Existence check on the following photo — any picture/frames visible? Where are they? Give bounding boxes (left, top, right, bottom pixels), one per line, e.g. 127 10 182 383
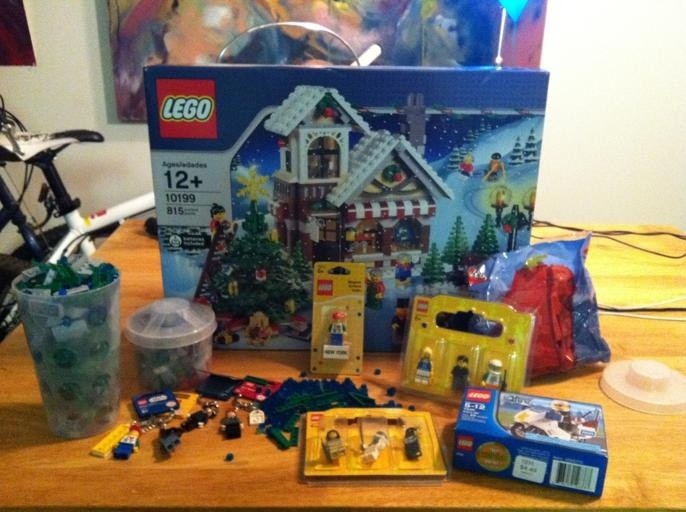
85 0 558 143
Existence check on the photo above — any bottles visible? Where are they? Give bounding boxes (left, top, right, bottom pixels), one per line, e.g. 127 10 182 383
127 295 217 392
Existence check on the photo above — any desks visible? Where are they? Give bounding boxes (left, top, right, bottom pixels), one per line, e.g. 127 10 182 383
0 215 685 511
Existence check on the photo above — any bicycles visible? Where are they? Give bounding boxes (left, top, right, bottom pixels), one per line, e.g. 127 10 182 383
0 21 383 343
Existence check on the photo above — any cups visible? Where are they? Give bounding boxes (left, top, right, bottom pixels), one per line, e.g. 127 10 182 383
8 261 122 438
